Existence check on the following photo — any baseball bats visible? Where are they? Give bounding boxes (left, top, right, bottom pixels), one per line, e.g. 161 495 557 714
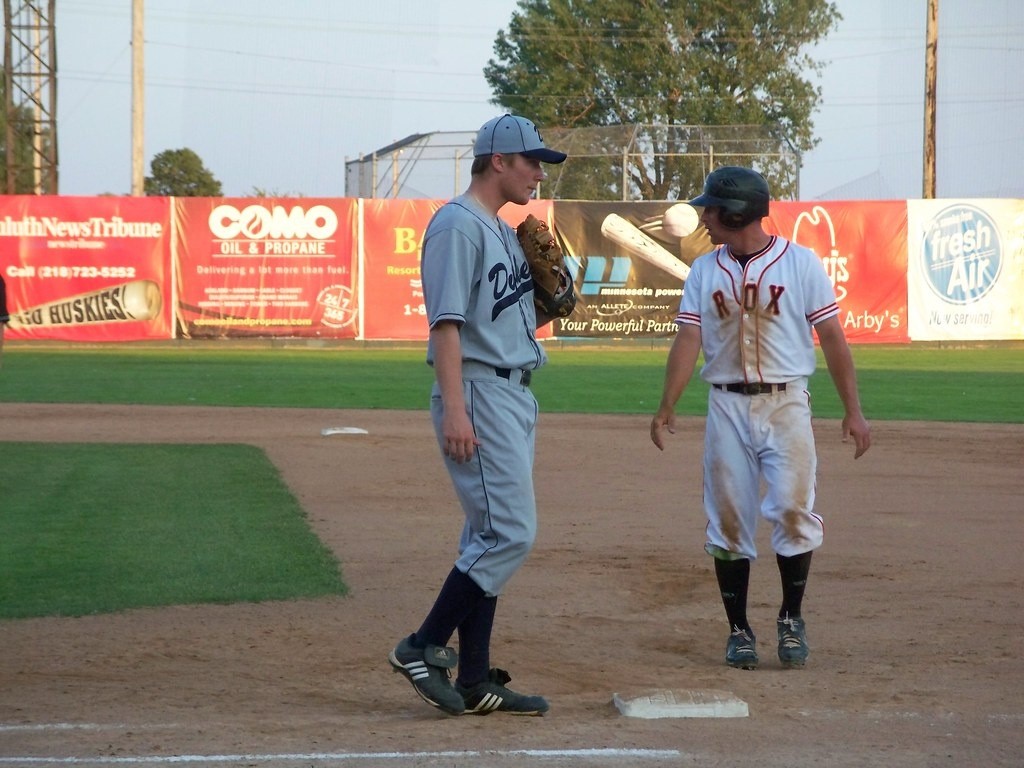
600 213 691 281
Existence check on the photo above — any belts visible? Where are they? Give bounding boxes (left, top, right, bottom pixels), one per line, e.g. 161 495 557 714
491 365 535 389
710 382 788 397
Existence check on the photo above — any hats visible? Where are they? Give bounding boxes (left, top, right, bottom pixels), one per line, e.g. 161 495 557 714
471 114 570 165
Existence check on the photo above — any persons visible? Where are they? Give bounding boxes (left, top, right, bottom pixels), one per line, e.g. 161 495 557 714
648 167 873 668
388 116 577 714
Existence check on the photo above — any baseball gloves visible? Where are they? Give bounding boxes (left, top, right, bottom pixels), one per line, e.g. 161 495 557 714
516 212 577 317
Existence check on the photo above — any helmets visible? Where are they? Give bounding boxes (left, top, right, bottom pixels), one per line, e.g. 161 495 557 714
686 166 774 228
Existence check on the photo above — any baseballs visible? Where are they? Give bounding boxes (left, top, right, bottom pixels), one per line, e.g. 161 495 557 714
662 202 699 237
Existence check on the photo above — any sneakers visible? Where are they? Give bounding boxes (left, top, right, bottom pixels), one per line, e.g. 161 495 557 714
449 666 552 715
724 623 769 671
387 633 488 713
774 611 810 663
